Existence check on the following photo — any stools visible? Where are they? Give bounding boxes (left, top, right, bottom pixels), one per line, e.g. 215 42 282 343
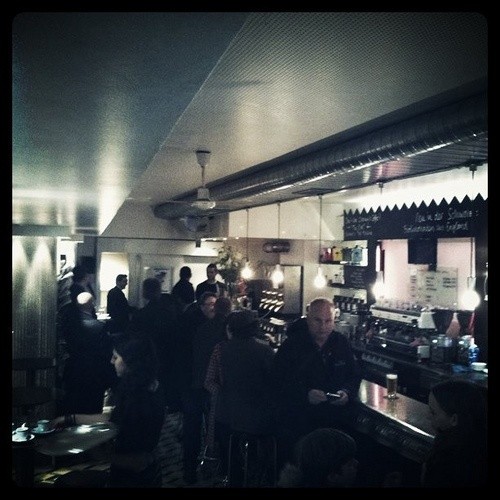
227 432 278 489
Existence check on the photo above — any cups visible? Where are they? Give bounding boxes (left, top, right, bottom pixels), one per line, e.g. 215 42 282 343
386 374 398 399
436 337 449 362
37 420 50 431
12 427 29 440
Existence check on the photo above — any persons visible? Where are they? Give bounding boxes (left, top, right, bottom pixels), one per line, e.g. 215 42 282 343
47 333 164 486
65 265 105 334
61 291 112 414
107 261 487 489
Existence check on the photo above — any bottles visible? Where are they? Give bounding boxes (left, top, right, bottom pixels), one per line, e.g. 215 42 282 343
357 300 364 315
380 319 394 340
333 295 342 309
361 318 380 336
346 297 353 312
394 321 417 344
351 298 359 315
341 297 348 312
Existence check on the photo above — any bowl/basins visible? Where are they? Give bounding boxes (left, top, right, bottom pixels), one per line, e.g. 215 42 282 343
472 362 487 371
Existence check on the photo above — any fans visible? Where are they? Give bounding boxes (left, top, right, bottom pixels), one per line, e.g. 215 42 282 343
123 150 257 212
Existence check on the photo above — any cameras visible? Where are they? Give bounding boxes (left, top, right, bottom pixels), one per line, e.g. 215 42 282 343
325 393 341 400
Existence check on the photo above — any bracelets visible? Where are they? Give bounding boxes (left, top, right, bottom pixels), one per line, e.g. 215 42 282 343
65 413 78 426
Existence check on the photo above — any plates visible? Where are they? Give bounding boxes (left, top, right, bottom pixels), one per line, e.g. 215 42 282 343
12 434 35 442
31 427 56 434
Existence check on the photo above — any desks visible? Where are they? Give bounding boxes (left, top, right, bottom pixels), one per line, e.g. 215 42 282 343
13 357 61 407
11 423 119 487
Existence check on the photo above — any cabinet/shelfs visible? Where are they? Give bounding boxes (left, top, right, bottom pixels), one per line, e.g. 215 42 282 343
317 259 361 288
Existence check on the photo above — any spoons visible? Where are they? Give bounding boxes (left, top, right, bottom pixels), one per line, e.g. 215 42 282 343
26 434 31 442
